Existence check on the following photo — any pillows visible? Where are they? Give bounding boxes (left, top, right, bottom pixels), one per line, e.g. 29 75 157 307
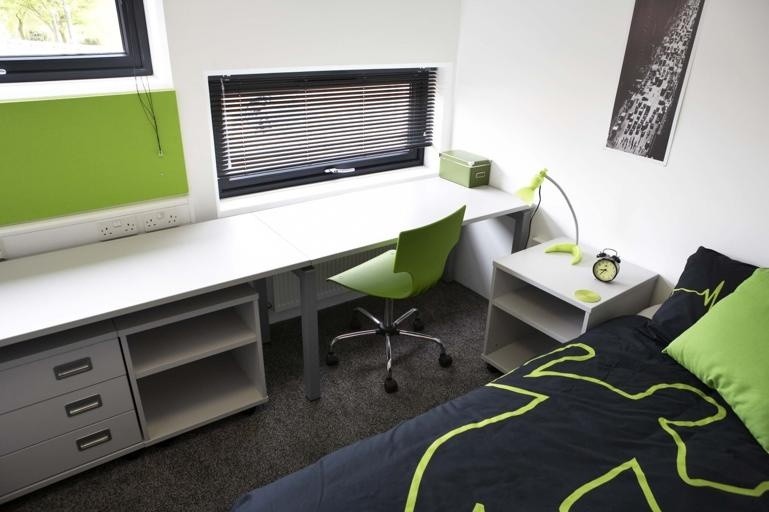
662 267 768 456
645 249 755 344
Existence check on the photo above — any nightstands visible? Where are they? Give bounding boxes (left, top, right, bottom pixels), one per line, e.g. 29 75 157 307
481 237 659 374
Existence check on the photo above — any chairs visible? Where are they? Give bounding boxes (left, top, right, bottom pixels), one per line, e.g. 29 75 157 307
327 204 466 394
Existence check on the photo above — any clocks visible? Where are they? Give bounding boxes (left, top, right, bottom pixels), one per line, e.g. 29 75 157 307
593 248 620 283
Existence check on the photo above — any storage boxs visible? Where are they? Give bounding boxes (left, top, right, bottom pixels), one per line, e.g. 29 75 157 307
439 150 490 188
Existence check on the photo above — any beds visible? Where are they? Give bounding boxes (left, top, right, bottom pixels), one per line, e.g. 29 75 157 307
229 246 769 512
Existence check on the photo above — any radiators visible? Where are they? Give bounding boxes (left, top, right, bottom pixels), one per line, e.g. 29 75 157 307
268 243 397 314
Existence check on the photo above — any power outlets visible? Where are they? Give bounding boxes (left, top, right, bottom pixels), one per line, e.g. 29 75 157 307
98 215 138 242
143 207 181 233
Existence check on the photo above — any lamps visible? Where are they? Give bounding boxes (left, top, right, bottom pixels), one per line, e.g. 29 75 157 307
514 168 582 266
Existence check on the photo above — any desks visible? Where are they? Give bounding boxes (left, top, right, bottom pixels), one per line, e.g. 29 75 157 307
0 176 534 504
